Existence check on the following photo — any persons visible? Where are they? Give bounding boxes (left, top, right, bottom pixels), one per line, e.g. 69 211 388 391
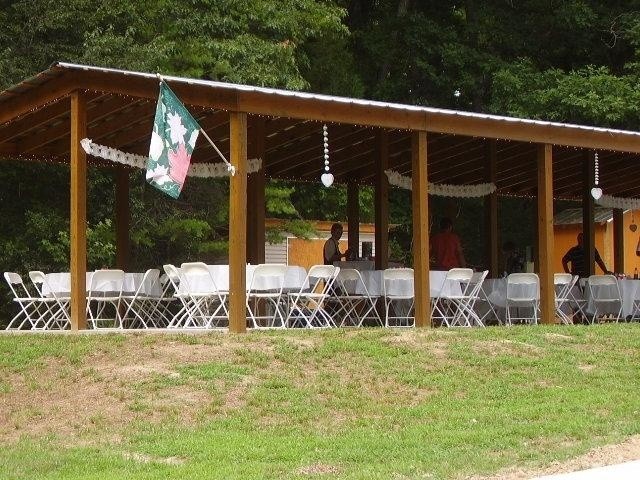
323 223 353 325
503 242 524 276
429 218 468 270
562 233 615 293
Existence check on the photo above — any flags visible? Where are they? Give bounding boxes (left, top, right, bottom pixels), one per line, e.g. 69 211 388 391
146 80 201 200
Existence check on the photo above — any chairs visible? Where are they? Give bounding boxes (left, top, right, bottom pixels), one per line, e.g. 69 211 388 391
246 262 415 328
3 262 73 330
432 269 542 325
85 263 231 329
553 274 638 325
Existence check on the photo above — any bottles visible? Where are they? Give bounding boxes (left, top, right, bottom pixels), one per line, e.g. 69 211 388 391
633 268 638 279
